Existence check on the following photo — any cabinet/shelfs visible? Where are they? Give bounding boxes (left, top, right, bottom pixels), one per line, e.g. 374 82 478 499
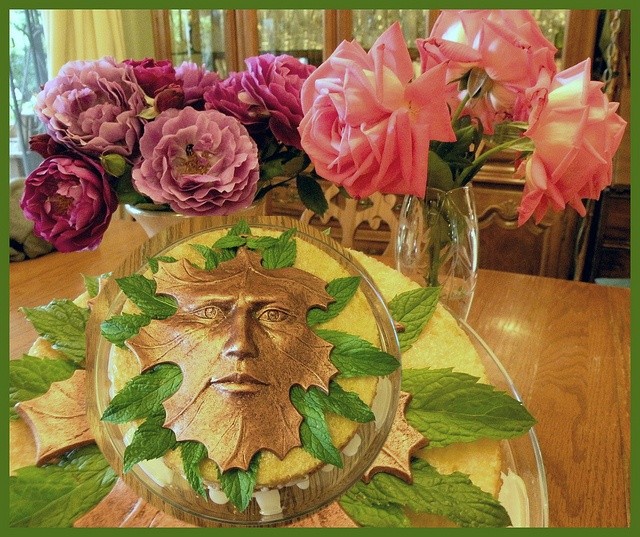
471 183 564 278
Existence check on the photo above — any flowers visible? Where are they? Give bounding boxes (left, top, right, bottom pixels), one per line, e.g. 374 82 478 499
295 10 629 292
18 53 318 254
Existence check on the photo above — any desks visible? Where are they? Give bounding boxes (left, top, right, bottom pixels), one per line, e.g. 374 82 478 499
10 205 629 525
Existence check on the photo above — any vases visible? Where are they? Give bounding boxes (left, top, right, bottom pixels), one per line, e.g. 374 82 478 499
123 202 267 241
395 186 479 328
